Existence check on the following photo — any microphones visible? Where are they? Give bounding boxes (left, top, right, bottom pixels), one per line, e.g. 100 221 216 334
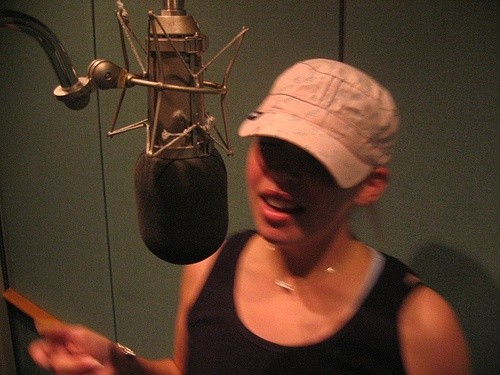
133 0 229 264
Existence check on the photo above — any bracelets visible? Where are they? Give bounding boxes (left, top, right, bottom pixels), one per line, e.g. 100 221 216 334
112 343 138 375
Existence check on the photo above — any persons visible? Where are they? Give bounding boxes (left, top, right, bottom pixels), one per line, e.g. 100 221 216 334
28 59 474 375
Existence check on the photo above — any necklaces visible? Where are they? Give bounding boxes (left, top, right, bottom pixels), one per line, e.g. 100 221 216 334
272 246 359 294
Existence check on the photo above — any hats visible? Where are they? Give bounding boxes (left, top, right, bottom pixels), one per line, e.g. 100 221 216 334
237 58 401 188
